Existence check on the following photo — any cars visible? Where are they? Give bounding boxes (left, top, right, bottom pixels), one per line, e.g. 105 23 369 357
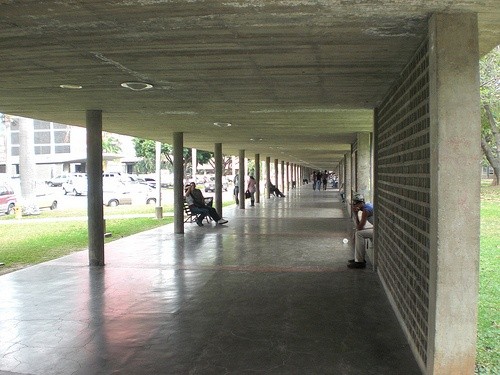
0 181 17 216
204 176 227 192
44 171 157 207
35 183 58 210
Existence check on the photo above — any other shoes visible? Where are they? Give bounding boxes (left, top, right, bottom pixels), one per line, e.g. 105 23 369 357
218 218 229 224
347 258 366 269
194 218 204 226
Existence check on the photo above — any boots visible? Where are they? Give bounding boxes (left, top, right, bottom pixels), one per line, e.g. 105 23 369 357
250 200 254 206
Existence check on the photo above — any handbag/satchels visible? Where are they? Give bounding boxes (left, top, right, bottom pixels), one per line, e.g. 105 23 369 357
245 190 251 199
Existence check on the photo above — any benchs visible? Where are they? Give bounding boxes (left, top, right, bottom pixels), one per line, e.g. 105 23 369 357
351 210 373 265
182 197 213 224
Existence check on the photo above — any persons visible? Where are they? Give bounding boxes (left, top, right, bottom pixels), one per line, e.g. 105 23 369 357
234 174 240 205
329 172 338 187
186 182 228 227
247 176 256 206
347 194 374 268
267 180 285 197
339 186 344 199
313 170 328 190
